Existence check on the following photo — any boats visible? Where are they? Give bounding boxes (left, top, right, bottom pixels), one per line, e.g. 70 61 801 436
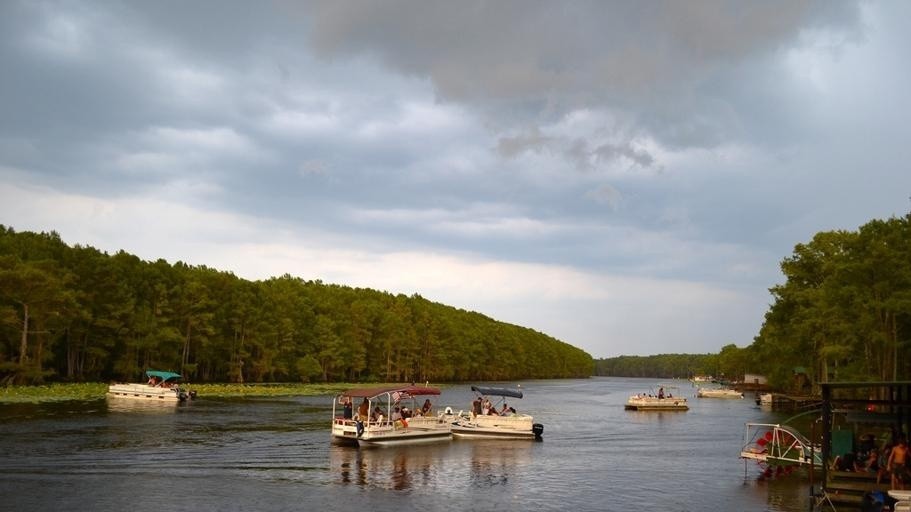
437 385 544 440
625 383 690 412
331 384 454 445
695 387 746 400
107 369 198 401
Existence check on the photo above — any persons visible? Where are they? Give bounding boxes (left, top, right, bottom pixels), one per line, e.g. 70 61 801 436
339 395 519 439
147 375 194 402
659 387 665 399
829 428 911 512
